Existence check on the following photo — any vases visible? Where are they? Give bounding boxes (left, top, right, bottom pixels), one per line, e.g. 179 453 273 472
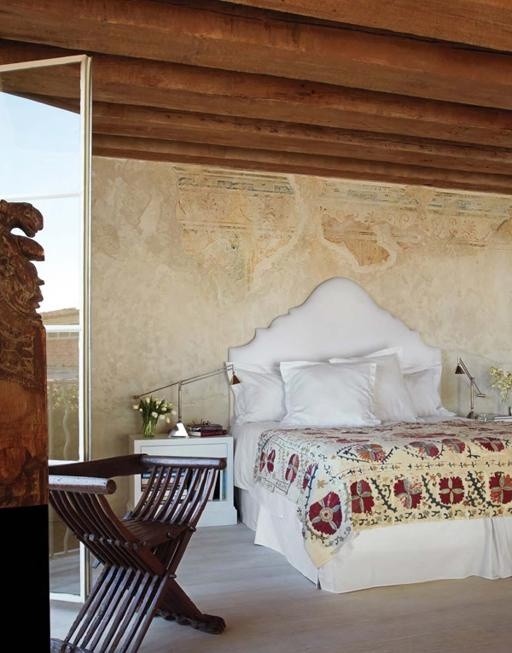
143 417 156 439
497 392 509 416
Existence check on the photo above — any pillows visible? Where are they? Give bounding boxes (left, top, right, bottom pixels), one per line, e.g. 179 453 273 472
277 358 382 429
329 345 418 428
226 362 286 425
402 362 457 424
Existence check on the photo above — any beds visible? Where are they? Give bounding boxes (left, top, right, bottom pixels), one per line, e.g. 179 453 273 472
226 276 512 596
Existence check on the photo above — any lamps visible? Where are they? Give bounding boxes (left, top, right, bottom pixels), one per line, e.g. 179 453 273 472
455 356 486 419
132 364 240 437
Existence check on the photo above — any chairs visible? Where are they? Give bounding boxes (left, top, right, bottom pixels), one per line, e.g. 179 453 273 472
46 452 227 653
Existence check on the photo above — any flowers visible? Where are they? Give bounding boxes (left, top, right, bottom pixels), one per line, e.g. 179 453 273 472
487 365 512 404
131 393 177 439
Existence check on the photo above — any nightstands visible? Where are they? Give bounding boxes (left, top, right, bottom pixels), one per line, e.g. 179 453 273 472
127 433 237 528
479 419 512 423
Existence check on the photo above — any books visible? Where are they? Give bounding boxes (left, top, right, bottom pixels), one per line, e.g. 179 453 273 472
188 425 228 437
142 468 219 505
